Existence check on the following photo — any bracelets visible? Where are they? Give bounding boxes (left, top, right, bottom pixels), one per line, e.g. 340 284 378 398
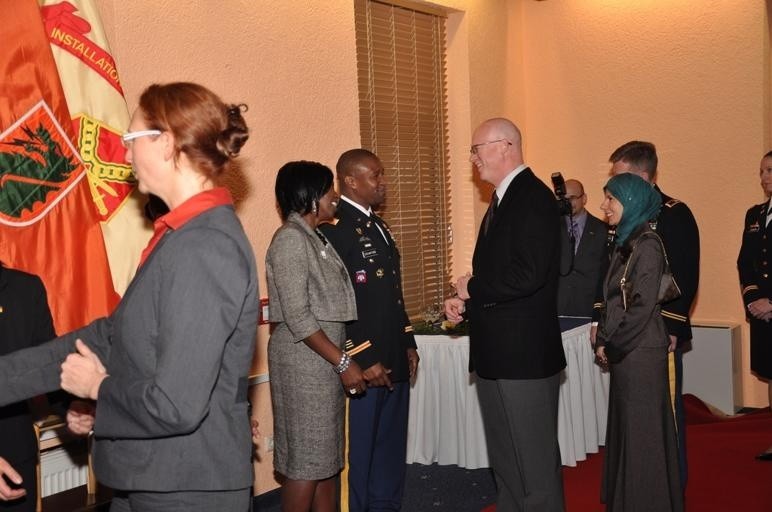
333 352 352 374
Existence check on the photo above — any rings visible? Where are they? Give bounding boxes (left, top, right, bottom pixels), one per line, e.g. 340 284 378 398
349 389 357 395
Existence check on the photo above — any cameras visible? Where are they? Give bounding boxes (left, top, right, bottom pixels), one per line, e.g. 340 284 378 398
551 172 574 215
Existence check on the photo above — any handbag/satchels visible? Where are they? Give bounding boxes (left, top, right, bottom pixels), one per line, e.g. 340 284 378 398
619 231 680 311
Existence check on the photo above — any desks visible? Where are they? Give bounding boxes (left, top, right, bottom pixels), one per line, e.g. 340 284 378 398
406 316 610 470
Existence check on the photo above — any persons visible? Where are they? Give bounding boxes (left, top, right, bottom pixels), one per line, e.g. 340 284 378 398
1 82 260 512
0 262 95 512
556 180 609 317
265 160 367 512
736 150 772 463
319 149 419 512
590 140 700 487
444 118 568 512
594 172 685 511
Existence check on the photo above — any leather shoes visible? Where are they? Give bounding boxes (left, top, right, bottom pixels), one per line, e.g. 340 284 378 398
756 448 772 460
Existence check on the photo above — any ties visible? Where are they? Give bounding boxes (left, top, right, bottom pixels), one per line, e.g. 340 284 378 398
485 191 498 234
369 213 388 246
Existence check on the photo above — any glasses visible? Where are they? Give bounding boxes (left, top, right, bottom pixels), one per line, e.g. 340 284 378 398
470 138 512 155
570 194 583 202
121 130 160 149
